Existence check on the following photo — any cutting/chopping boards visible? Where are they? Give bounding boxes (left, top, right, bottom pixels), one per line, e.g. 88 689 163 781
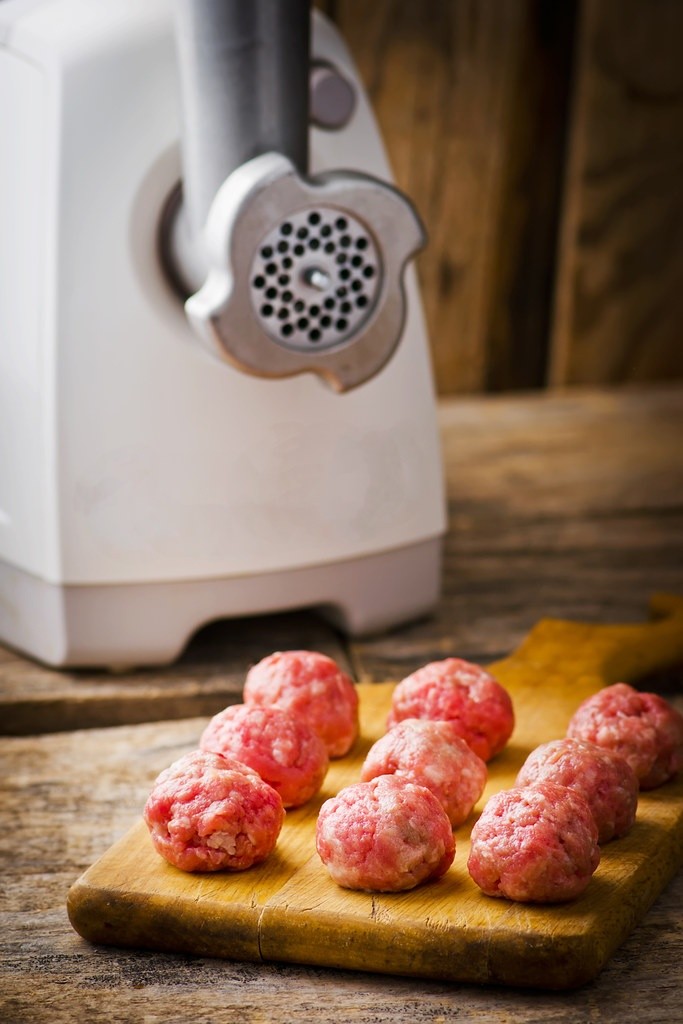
71 591 683 989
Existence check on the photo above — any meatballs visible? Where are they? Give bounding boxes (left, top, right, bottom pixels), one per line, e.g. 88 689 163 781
143 649 683 903
